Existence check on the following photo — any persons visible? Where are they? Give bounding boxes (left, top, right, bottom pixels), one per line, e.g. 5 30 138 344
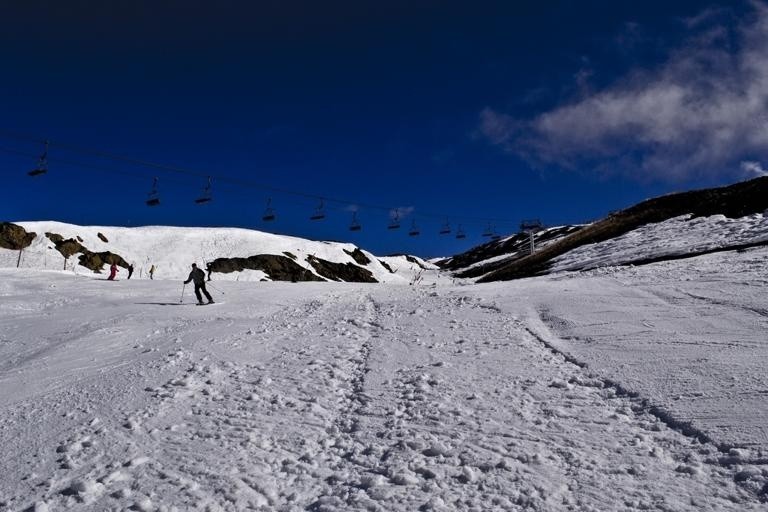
107 261 119 280
127 264 134 279
204 262 212 281
149 264 156 280
183 263 215 305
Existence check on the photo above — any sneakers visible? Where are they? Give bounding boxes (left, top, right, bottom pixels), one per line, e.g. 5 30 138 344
208 299 213 303
199 300 204 304
